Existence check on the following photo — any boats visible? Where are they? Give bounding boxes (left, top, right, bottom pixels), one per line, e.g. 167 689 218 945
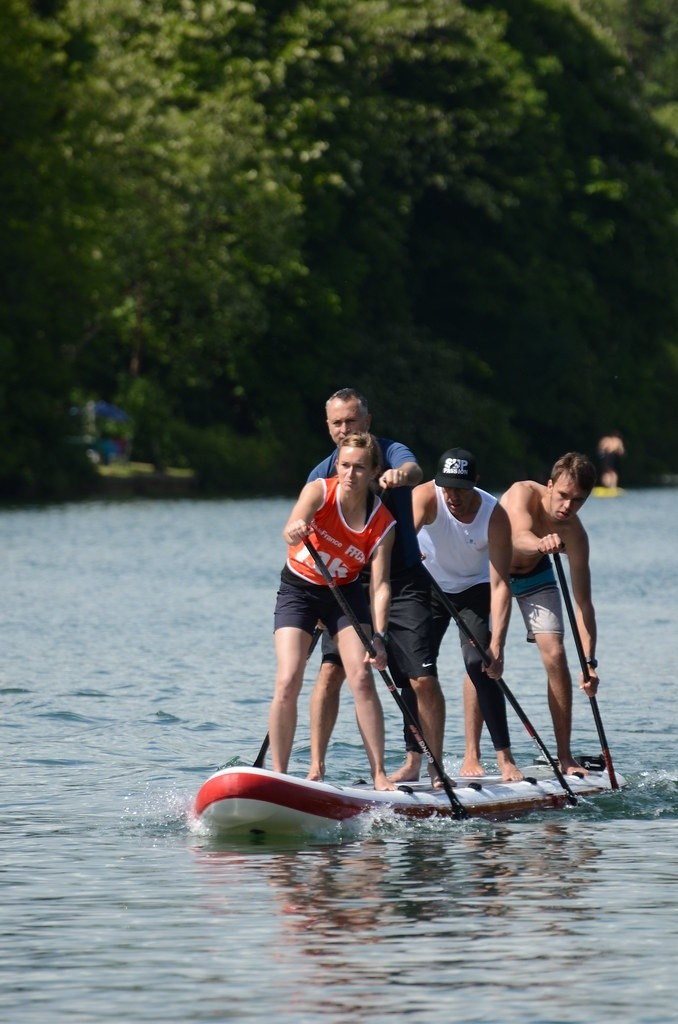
192 755 628 838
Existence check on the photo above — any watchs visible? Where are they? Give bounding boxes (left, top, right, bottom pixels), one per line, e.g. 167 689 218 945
373 633 388 645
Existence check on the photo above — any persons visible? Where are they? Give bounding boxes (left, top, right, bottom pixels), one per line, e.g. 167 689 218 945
388 447 525 782
460 451 599 776
269 432 397 790
600 436 625 488
306 388 457 788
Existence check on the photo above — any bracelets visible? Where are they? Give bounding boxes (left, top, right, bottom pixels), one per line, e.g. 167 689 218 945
585 656 598 669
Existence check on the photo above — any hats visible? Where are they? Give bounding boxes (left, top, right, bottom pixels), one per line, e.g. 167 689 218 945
436 446 477 490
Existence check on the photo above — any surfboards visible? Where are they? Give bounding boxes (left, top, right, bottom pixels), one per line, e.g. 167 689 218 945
195 756 628 827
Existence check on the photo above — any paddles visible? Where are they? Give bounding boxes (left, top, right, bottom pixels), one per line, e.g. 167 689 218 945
419 562 580 807
537 539 618 790
300 533 472 820
253 485 393 768
